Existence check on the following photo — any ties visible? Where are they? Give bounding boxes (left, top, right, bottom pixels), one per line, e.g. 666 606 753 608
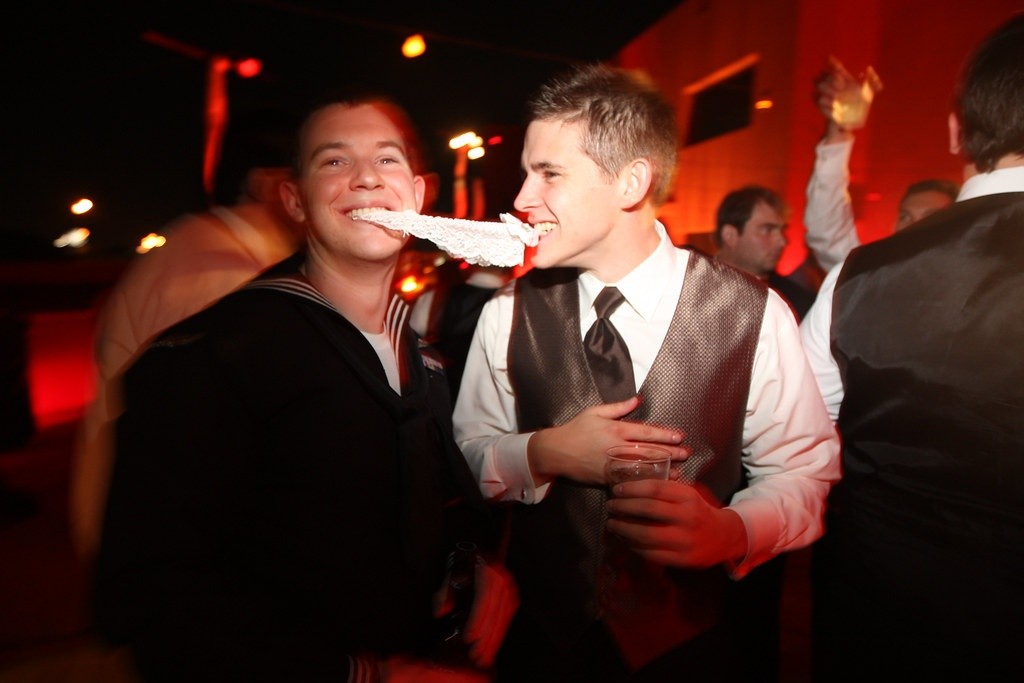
583 287 636 405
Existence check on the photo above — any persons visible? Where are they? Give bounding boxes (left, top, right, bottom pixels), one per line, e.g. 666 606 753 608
809 13 1023 682
62 90 522 683
450 61 844 682
798 63 956 419
712 185 814 323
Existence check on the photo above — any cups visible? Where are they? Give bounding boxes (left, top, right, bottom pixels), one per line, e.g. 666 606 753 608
832 76 875 130
606 445 673 497
